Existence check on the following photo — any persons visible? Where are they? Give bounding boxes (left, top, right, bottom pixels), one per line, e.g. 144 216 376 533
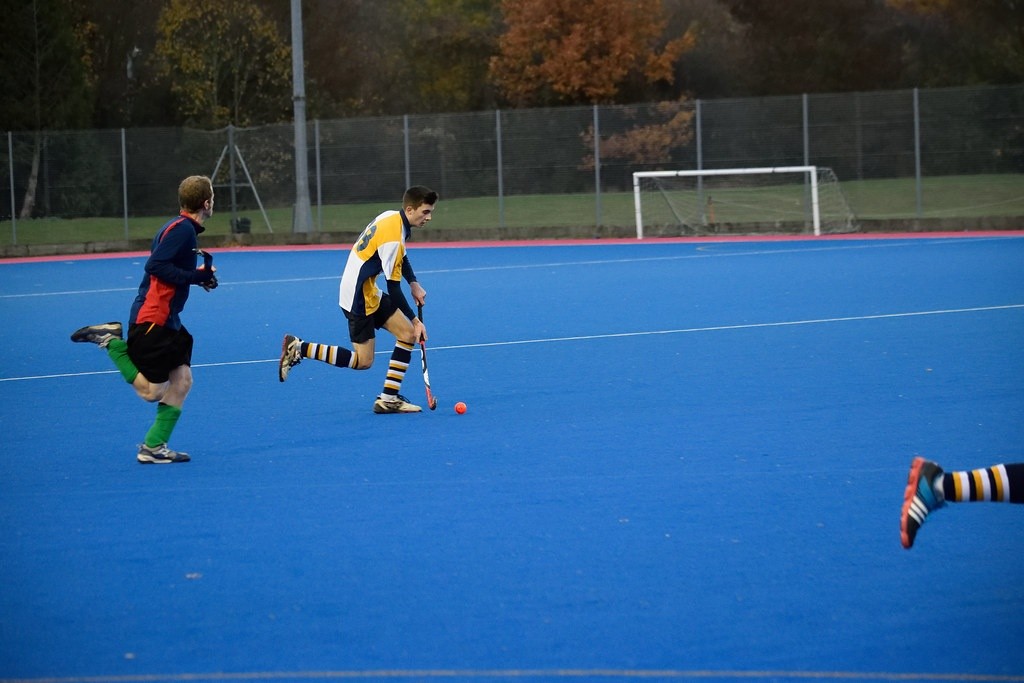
279 185 439 414
899 456 1024 549
70 175 218 463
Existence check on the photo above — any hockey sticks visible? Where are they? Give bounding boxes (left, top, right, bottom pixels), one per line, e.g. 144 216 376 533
416 303 438 410
191 248 214 293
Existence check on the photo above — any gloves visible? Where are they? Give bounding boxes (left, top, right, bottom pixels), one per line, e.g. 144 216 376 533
201 270 219 292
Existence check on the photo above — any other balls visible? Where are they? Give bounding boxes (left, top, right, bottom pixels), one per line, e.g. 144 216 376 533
453 401 468 415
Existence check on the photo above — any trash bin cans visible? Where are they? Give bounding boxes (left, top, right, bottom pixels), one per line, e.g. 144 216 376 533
229 216 251 234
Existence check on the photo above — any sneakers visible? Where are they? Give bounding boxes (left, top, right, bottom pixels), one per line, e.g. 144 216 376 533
71 321 123 349
373 394 422 413
278 334 302 383
900 455 948 550
136 444 190 464
